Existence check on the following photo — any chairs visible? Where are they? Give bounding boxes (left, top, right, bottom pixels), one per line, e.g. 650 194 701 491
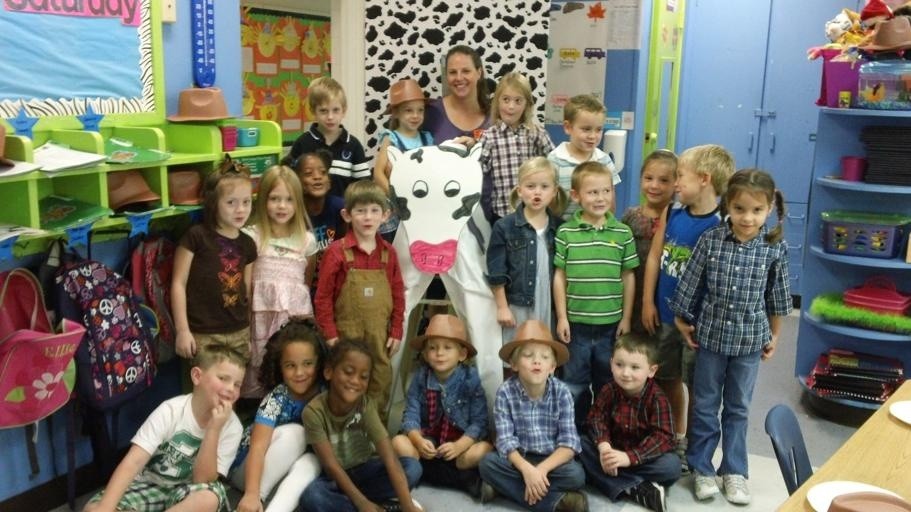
765 404 811 496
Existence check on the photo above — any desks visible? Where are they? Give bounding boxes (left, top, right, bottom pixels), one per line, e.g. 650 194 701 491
774 378 911 512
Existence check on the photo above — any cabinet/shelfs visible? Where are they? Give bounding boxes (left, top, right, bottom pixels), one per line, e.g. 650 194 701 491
794 105 910 410
0 116 284 245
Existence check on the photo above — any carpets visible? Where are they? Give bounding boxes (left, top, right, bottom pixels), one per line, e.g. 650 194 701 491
410 442 817 512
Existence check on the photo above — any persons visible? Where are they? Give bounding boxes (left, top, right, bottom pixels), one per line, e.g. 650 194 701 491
578 330 682 511
480 321 589 511
239 166 319 399
667 168 795 505
287 46 680 425
294 335 424 511
641 144 735 476
84 339 252 511
171 154 257 397
228 314 337 512
392 313 493 497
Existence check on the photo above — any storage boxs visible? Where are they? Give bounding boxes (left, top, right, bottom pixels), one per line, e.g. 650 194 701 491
822 59 868 108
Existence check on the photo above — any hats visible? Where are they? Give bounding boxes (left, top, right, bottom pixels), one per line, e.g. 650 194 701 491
382 80 439 117
105 165 205 218
0 124 15 168
856 17 911 54
164 87 239 122
498 318 571 368
408 312 478 360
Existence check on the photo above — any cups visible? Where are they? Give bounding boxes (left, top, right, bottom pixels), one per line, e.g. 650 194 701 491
843 157 867 181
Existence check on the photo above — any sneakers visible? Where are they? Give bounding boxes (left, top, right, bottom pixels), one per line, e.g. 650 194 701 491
482 484 505 504
724 475 753 506
557 491 591 511
624 477 667 511
693 467 722 502
674 433 689 473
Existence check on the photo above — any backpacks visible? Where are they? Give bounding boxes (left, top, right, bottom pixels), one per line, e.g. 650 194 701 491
1 234 178 430
